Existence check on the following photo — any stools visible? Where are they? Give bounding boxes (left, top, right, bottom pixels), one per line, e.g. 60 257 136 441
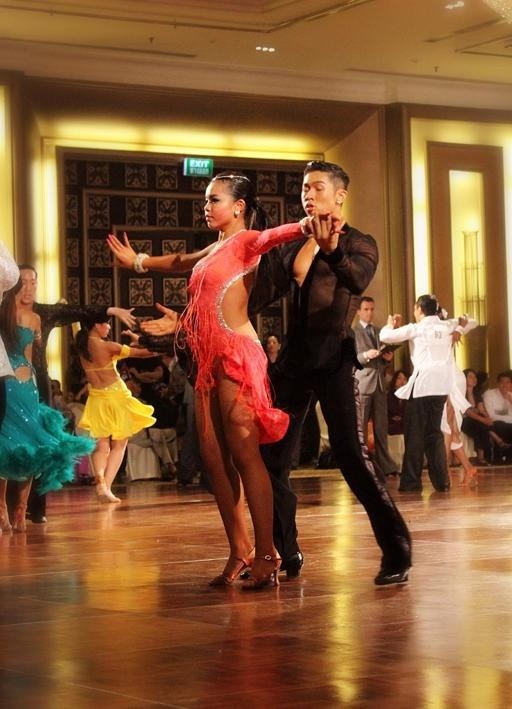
386 432 429 473
458 431 479 460
125 441 164 483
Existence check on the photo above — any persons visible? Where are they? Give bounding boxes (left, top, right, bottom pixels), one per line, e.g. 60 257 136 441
459 369 511 465
261 335 286 392
55 380 90 434
139 161 414 588
378 295 478 493
106 172 345 591
484 372 512 465
1 237 24 437
118 360 138 398
2 265 138 524
2 277 98 533
390 370 410 436
351 296 404 479
397 304 480 488
137 334 169 483
54 298 170 505
169 336 204 484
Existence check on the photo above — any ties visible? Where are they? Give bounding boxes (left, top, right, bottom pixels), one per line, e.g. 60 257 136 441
366 324 376 348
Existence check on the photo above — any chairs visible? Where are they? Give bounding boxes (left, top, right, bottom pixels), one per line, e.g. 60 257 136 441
64 400 113 481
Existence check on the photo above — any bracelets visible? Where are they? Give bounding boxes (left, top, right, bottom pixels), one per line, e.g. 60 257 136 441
134 253 151 275
299 216 317 238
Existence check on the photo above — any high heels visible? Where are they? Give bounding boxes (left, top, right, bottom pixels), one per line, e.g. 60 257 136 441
459 467 479 487
12 504 28 532
450 432 464 451
0 505 13 535
242 552 284 590
210 546 255 586
96 476 122 503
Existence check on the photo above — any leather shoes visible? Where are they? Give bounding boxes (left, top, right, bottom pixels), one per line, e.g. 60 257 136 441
498 441 512 451
31 514 47 523
241 551 304 578
399 487 422 492
375 566 410 585
477 458 491 466
435 481 450 492
